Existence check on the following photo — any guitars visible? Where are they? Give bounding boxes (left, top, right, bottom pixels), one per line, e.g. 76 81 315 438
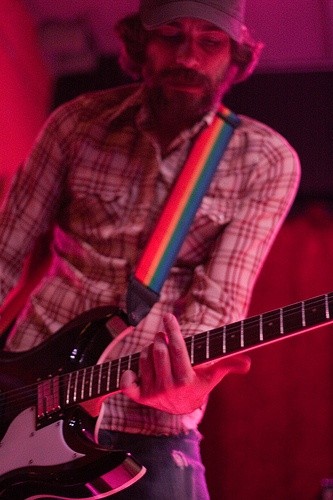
1 292 332 500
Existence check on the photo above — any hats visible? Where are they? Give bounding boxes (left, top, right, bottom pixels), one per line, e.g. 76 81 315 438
137 0 247 42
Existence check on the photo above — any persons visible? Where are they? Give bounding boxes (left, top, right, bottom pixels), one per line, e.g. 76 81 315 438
0 0 305 500
